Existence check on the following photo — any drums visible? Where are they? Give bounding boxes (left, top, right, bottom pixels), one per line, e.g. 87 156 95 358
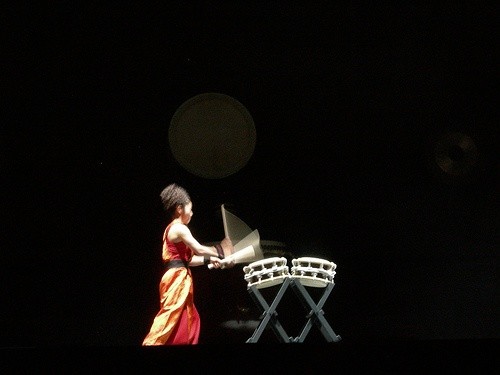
291 256 337 287
244 257 290 288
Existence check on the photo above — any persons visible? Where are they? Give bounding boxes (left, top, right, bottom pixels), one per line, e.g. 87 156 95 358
142 182 233 345
